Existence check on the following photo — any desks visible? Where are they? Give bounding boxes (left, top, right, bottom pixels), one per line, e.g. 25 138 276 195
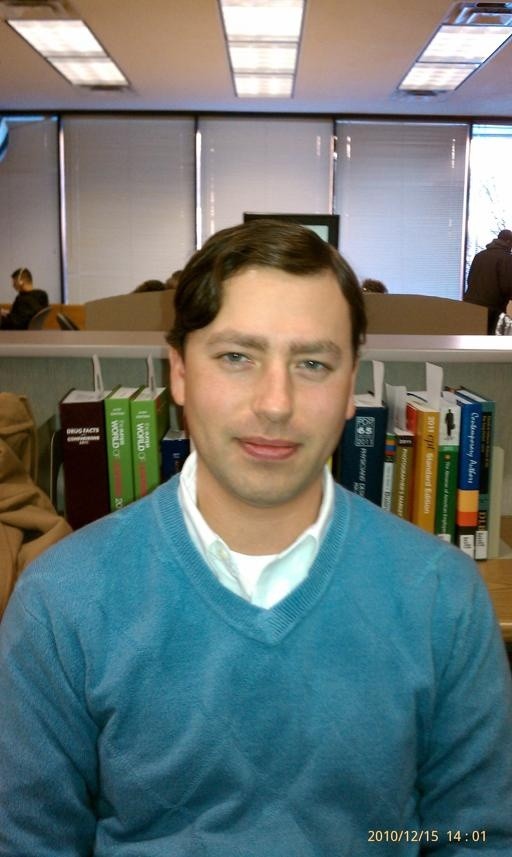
0 331 512 642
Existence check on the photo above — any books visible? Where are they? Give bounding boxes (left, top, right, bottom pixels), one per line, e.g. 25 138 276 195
329 387 494 562
59 384 191 533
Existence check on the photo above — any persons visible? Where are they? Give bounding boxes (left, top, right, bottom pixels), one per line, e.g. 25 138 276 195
1 216 512 856
1 266 52 330
128 269 183 295
363 278 387 294
463 228 512 335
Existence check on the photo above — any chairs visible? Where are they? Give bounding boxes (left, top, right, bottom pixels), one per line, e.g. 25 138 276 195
28 307 53 330
57 312 80 330
